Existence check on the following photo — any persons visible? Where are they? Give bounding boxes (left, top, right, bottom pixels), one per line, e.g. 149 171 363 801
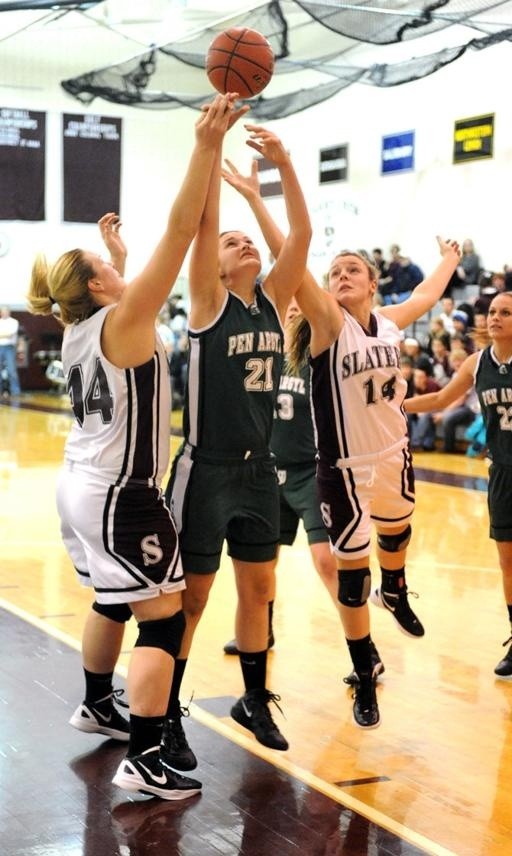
371 234 511 291
160 294 190 407
0 306 26 400
401 292 511 676
403 395 482 453
159 92 317 770
26 90 231 801
222 293 386 685
218 158 463 730
402 292 494 394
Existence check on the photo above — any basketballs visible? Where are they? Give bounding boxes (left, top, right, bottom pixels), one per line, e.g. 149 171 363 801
209 29 276 99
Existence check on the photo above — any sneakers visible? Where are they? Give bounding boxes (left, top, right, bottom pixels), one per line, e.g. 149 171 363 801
156 692 198 771
69 689 131 741
224 630 275 655
343 655 384 686
494 637 511 680
231 689 289 751
112 746 202 801
370 584 424 638
351 673 380 729
